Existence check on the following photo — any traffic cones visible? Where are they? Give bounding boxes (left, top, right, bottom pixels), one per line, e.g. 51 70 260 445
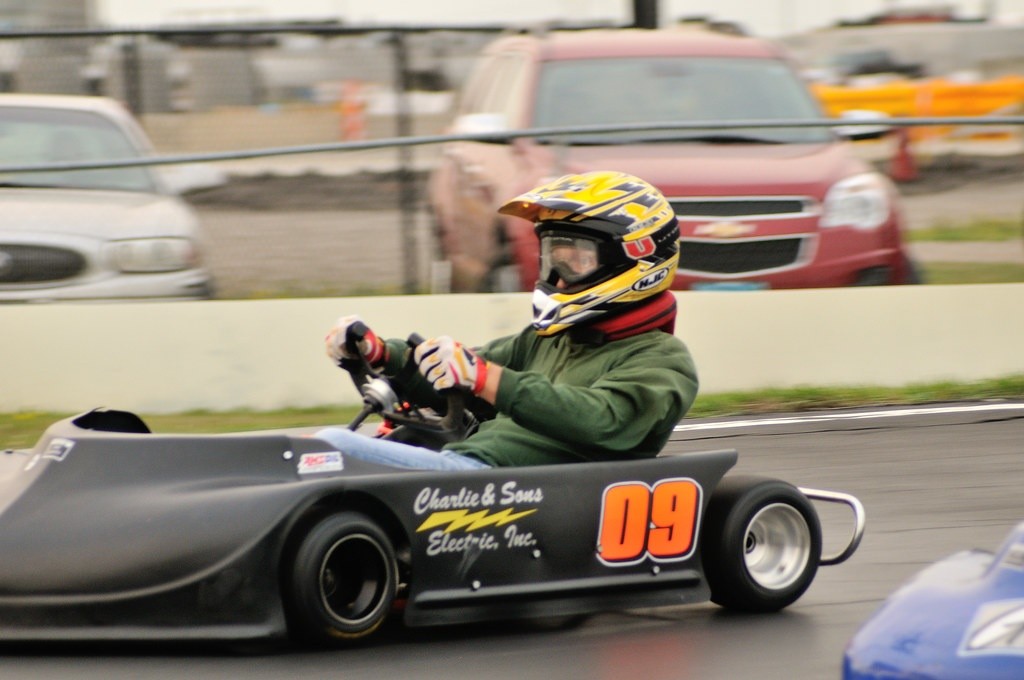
335 75 370 141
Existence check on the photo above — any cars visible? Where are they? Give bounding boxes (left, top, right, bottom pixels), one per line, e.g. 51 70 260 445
1 92 217 304
421 26 920 293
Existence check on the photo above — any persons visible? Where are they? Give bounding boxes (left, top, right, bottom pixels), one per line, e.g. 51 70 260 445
326 172 699 471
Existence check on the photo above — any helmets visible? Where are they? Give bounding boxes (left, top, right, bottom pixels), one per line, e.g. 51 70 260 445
497 170 682 338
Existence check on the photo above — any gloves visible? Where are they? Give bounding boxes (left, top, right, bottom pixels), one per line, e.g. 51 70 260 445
414 335 490 396
324 315 390 372
